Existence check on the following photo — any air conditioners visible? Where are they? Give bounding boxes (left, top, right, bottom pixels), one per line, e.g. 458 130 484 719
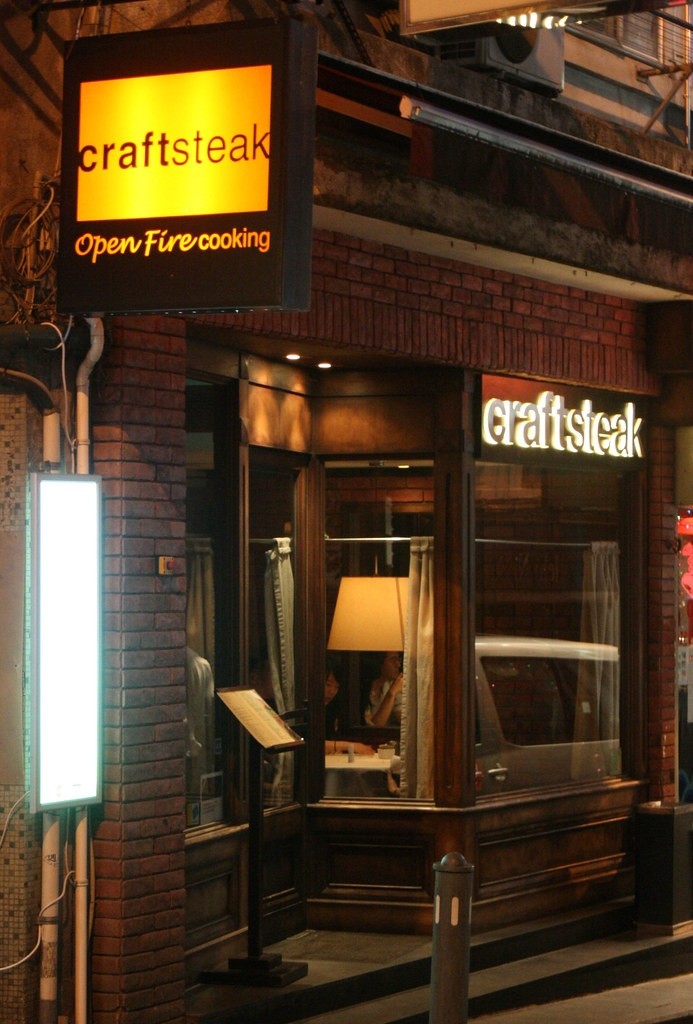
436 24 565 99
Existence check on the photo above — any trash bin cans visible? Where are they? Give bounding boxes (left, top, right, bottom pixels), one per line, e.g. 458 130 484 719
636 799 693 937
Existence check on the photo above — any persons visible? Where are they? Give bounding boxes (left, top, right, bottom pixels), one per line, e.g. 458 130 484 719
324 657 375 756
362 651 403 728
249 657 274 706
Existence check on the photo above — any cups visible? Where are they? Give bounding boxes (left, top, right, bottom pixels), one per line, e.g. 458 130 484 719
348 744 354 754
378 748 395 759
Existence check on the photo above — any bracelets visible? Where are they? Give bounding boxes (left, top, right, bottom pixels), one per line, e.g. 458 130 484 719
334 740 336 752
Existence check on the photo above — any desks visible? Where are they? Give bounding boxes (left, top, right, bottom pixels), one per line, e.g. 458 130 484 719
325 754 400 797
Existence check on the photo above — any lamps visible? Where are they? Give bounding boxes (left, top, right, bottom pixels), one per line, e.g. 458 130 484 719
327 554 409 651
30 471 104 816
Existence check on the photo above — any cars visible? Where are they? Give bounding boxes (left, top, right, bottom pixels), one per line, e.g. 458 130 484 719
473 637 618 796
